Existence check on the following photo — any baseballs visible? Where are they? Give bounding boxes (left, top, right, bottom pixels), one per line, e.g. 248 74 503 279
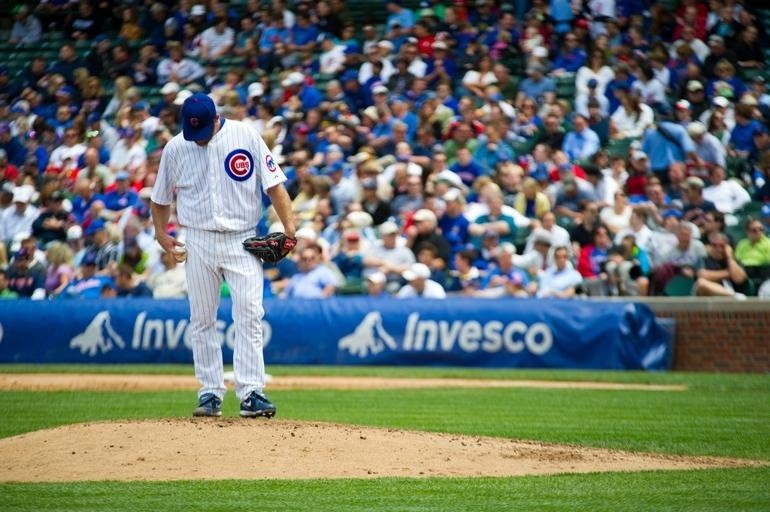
174 248 186 262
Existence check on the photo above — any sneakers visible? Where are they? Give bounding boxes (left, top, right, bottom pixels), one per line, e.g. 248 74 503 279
193 394 223 416
240 391 276 419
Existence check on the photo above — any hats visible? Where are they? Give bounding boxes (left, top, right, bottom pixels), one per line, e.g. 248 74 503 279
0 0 770 301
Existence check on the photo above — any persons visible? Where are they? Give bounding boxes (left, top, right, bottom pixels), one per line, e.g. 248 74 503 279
151 92 297 418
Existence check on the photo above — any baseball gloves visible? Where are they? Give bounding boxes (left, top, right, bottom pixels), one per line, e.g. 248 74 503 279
242 233 297 265
